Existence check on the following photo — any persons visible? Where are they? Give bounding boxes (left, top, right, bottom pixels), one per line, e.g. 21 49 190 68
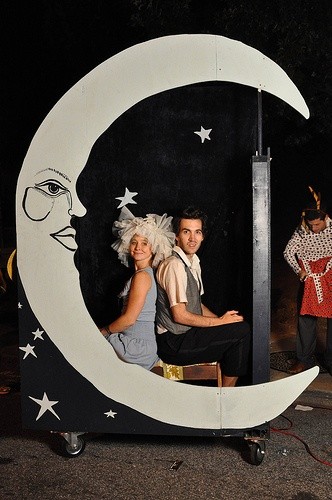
96 205 177 371
283 209 332 374
155 206 253 387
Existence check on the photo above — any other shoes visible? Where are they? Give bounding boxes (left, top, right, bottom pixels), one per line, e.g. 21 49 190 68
289 362 304 374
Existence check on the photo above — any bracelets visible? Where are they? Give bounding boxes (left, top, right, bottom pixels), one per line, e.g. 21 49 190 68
105 327 113 335
